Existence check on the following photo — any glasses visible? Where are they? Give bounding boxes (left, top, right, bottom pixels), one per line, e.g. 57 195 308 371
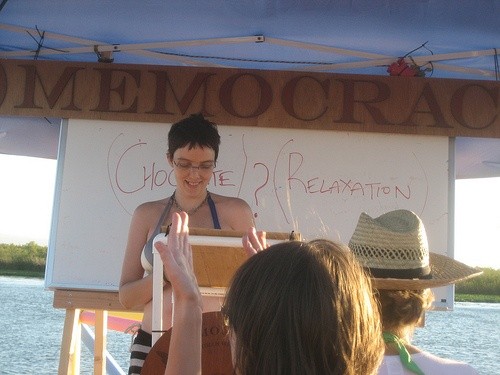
174 161 216 172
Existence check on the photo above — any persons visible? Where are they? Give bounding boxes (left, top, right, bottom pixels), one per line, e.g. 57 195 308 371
154 212 385 374
117 114 260 375
347 209 486 374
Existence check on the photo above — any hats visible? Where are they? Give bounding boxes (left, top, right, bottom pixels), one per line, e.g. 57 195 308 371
348 209 485 290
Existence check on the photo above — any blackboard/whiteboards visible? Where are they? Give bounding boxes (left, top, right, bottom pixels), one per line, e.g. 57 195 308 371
45 115 459 316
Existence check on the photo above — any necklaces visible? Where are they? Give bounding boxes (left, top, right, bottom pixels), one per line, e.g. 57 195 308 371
174 195 208 215
